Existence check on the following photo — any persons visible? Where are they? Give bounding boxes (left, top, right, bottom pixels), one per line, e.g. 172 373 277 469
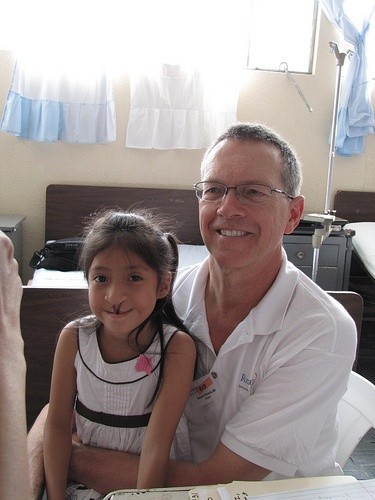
43 212 195 500
26 124 357 500
0 232 31 500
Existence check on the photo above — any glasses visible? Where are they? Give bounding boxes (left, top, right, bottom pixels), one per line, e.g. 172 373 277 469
192 181 295 204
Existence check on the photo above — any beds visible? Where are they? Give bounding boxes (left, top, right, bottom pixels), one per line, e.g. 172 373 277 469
333 191 375 283
20 184 364 435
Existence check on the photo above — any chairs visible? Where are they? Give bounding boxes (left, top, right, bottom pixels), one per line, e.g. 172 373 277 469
261 370 374 480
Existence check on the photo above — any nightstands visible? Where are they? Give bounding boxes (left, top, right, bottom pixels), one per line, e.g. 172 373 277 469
282 227 356 291
0 215 27 279
